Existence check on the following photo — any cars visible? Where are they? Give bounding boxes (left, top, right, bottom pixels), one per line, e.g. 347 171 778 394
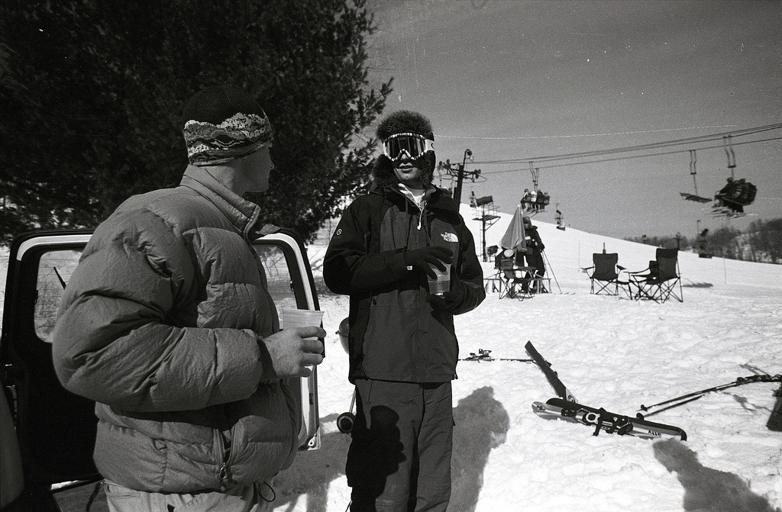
1 226 322 511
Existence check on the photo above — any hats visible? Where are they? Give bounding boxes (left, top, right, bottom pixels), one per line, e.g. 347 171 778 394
182 87 274 166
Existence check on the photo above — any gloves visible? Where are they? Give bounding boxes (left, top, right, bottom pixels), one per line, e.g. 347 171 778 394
429 279 467 312
403 247 453 281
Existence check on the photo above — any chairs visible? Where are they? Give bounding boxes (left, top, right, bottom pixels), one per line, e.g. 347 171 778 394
485 246 683 304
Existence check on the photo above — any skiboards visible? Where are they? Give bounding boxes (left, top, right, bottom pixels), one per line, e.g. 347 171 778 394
518 289 575 296
697 206 758 222
525 209 539 218
525 340 687 443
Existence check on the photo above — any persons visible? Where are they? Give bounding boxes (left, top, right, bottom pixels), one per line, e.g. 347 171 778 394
503 250 532 293
323 111 486 512
711 177 757 217
52 85 326 512
520 188 550 212
521 216 545 293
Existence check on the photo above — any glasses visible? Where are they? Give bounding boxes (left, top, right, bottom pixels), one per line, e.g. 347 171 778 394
382 134 435 161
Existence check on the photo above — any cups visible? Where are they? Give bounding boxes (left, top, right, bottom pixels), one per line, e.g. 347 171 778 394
425 256 452 297
281 308 324 373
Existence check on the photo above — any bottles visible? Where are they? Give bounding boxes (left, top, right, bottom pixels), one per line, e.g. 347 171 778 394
603 242 606 254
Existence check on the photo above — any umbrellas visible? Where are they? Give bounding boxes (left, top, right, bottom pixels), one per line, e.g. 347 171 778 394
500 205 525 252
